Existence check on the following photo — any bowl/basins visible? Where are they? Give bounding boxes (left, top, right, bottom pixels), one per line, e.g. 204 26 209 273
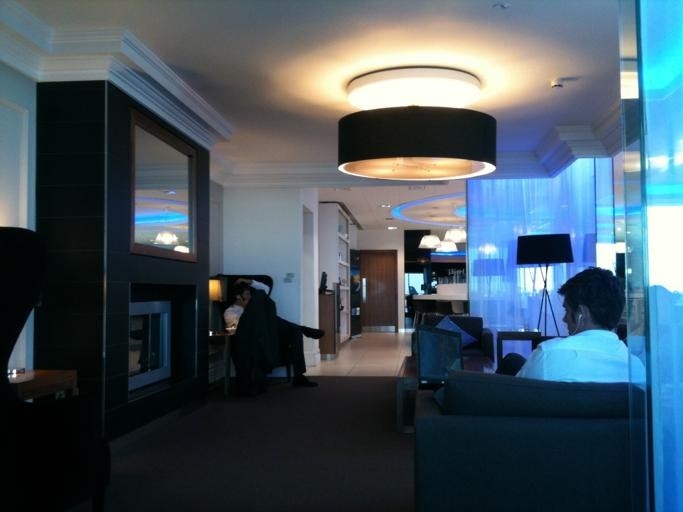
225 327 236 334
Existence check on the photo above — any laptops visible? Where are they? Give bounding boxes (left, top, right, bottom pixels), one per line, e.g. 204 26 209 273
415 323 468 392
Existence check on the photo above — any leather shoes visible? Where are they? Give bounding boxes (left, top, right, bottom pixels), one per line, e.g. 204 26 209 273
292 376 318 387
306 329 324 339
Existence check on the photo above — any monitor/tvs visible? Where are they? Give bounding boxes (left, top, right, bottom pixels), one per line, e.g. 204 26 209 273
319 272 327 292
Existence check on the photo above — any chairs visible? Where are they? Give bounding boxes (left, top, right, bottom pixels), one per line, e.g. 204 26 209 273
209 275 292 397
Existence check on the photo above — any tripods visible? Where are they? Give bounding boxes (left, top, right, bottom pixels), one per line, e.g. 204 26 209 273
533 289 561 340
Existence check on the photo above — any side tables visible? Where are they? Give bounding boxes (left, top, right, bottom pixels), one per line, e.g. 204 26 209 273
1 366 79 405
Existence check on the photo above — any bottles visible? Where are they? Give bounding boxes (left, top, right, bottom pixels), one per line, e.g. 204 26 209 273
431 271 438 294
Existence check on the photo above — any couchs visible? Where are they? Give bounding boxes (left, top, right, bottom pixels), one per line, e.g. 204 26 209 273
413 314 494 367
413 372 652 510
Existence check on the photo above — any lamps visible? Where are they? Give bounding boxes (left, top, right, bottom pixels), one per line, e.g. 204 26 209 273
515 234 574 336
417 228 467 253
208 279 223 303
336 66 496 182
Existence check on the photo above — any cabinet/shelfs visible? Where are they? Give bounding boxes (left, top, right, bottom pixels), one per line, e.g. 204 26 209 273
319 202 351 346
319 283 341 359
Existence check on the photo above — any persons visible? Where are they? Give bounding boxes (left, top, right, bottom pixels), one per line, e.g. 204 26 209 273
501 267 644 382
222 281 324 388
